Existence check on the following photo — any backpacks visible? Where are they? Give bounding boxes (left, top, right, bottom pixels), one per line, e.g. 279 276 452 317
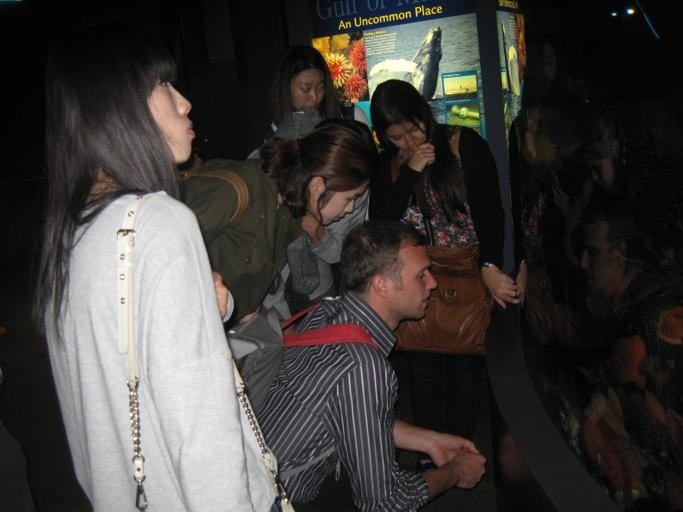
226 298 382 413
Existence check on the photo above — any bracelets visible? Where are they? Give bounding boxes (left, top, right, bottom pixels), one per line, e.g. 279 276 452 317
482 260 495 267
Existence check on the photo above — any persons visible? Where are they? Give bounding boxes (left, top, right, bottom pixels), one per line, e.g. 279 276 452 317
372 79 523 474
30 23 296 512
248 107 371 314
259 43 369 148
179 116 381 331
255 215 486 512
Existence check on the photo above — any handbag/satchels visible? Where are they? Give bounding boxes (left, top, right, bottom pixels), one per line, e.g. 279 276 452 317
393 243 498 357
